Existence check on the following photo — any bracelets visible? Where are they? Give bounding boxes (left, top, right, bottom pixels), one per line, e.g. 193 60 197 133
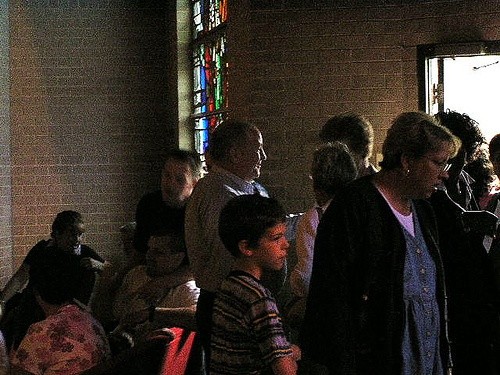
148 305 156 322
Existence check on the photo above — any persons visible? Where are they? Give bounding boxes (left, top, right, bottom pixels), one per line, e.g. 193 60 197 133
185 118 267 375
319 112 378 177
0 211 111 353
102 228 201 355
301 111 461 375
289 142 357 297
5 269 114 375
428 108 500 375
118 149 203 304
210 194 301 375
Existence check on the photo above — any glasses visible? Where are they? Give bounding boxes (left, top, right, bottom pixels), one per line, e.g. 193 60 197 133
412 154 452 172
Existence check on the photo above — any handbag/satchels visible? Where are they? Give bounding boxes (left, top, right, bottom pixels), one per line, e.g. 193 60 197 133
282 292 308 326
